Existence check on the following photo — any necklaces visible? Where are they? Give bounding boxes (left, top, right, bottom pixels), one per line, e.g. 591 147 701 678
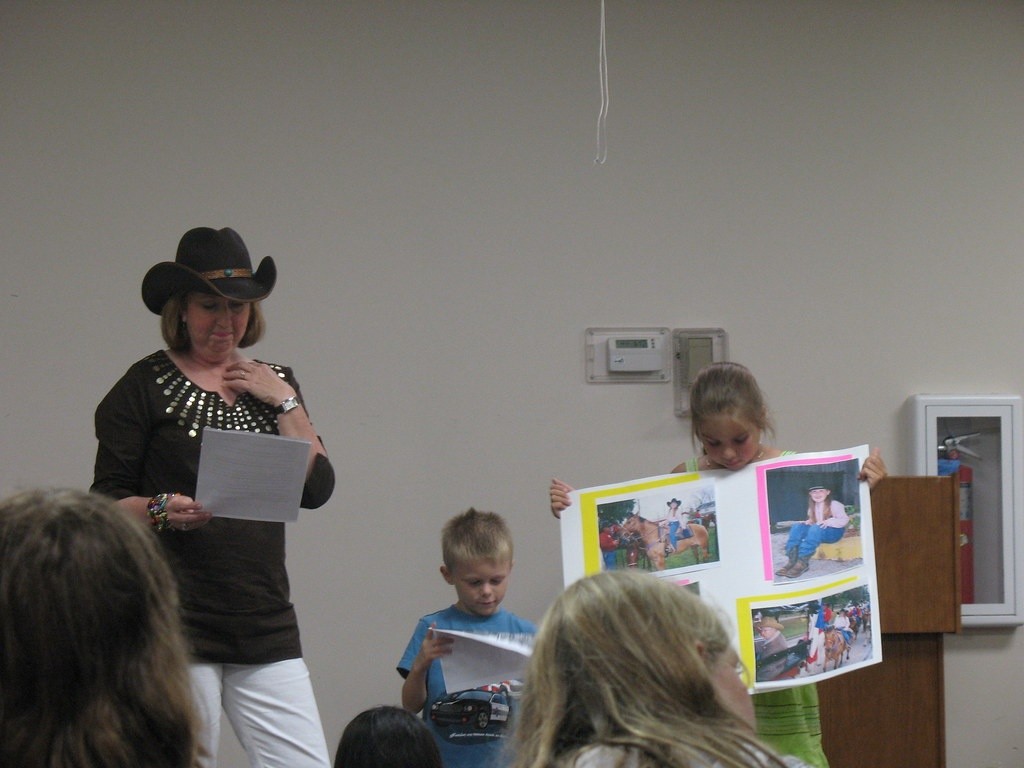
705 442 765 469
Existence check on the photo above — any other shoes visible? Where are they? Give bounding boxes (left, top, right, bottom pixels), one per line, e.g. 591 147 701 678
665 549 675 553
786 556 809 578
776 546 797 575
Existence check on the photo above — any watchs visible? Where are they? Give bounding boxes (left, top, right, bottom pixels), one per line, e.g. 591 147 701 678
275 394 303 415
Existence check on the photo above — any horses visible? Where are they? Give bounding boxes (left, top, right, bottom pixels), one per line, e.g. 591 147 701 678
824 611 870 671
617 512 709 571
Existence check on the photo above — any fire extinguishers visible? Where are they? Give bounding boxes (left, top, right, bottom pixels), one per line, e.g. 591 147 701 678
937 431 983 605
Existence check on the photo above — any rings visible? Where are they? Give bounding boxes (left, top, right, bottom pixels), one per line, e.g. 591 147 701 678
240 370 249 379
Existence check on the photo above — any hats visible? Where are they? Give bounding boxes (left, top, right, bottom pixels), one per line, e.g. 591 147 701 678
753 617 784 631
142 227 276 318
602 522 612 527
667 498 681 506
808 478 834 490
837 609 848 614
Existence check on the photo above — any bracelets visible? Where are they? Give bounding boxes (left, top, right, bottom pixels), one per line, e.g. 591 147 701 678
145 492 181 535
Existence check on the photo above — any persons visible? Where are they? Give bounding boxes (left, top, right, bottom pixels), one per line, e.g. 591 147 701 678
86 226 335 768
754 616 787 681
0 487 214 768
818 595 871 655
549 358 887 766
394 508 543 768
333 706 444 768
508 572 810 768
598 496 716 573
774 475 850 579
